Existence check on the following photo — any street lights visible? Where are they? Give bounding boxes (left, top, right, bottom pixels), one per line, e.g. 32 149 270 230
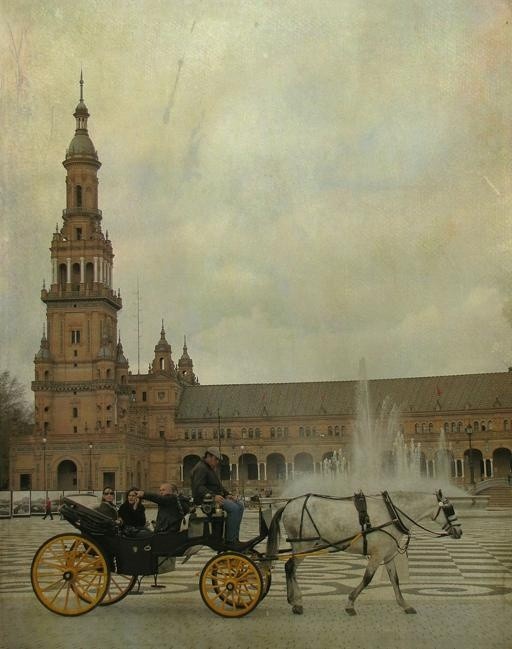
465 423 477 484
88 442 94 490
240 442 245 506
42 436 47 513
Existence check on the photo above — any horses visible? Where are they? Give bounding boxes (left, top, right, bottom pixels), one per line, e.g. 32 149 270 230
265 488 463 617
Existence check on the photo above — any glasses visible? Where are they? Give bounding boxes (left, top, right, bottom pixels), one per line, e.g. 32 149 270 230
104 492 113 495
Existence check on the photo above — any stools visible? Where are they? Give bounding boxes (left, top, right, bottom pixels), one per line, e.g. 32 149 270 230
190 503 228 539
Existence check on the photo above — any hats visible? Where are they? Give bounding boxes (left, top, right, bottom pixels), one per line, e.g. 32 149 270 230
208 447 223 460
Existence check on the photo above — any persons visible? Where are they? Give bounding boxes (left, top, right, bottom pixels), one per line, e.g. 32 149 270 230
118 487 151 536
260 487 272 498
191 446 244 545
42 497 53 520
100 486 124 529
134 482 189 534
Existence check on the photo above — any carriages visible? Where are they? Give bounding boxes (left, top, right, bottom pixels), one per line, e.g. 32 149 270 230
29 488 463 619
